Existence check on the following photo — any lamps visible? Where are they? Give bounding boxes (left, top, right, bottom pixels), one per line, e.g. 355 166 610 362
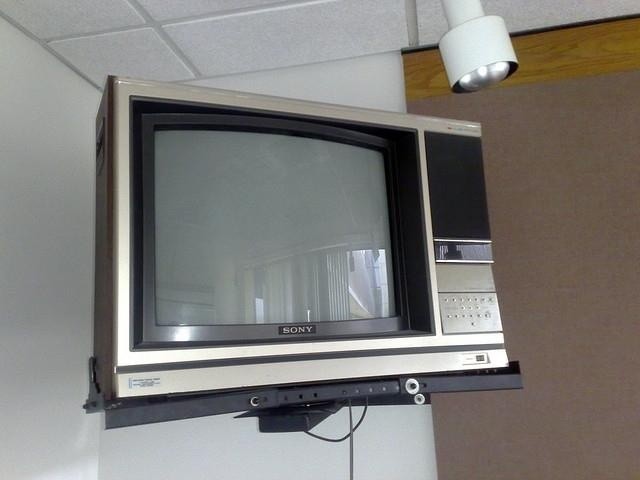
436 0 520 93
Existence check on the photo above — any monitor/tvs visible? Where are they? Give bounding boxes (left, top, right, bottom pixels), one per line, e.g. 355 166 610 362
94 74 511 404
